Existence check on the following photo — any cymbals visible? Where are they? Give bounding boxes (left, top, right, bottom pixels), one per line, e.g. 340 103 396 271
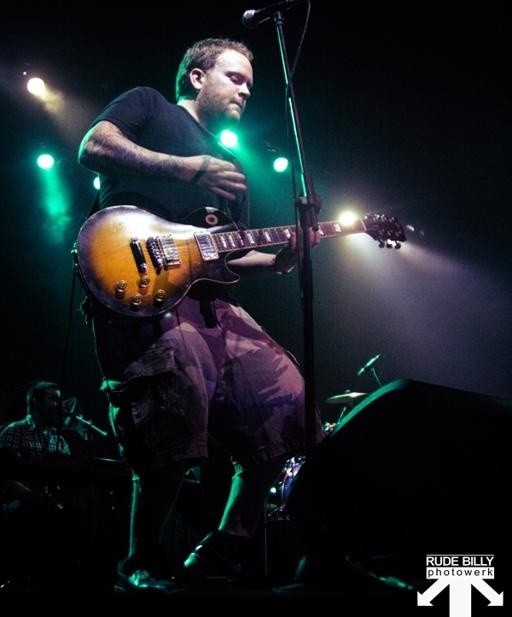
326 390 367 405
357 352 384 376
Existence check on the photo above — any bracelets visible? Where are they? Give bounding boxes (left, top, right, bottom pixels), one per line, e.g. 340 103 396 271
271 262 296 274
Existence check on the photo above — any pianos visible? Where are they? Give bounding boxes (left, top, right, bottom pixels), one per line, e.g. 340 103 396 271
0 445 132 489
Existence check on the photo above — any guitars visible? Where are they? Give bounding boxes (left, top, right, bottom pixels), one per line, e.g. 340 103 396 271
74 204 407 319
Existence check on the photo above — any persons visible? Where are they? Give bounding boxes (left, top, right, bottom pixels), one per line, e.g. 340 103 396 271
2 361 512 617
72 36 317 600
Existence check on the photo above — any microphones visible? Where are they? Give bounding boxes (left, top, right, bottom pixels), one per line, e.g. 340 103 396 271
242 0 301 26
